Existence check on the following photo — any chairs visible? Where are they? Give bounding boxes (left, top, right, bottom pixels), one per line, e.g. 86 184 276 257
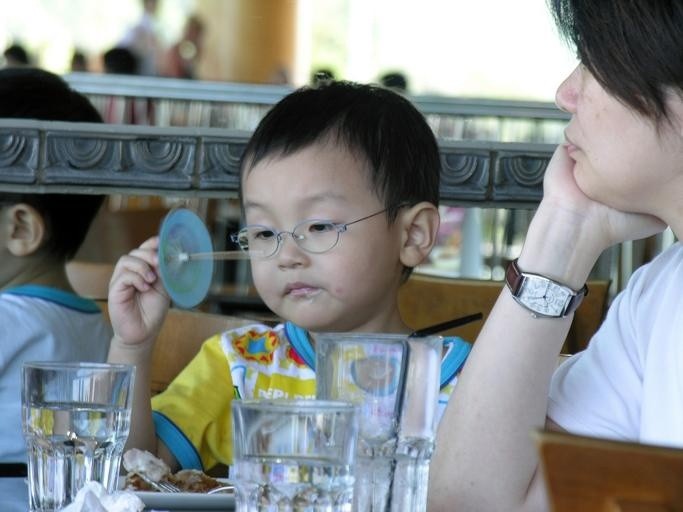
88 271 683 512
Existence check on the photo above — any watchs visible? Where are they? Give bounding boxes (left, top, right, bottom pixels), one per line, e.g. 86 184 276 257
504 258 589 319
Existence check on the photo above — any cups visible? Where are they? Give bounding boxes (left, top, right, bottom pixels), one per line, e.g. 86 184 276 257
230 321 444 508
22 358 137 505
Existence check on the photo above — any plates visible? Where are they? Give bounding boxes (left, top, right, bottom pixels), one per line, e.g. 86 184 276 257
130 466 230 507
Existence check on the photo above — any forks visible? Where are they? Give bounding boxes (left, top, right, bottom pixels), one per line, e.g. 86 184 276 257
140 470 232 493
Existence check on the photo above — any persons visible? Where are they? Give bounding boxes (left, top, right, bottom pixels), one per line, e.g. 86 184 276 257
4 0 205 78
0 67 110 512
106 82 472 474
429 0 683 512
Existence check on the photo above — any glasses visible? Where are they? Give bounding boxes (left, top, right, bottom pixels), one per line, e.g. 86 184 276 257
230 200 409 258
